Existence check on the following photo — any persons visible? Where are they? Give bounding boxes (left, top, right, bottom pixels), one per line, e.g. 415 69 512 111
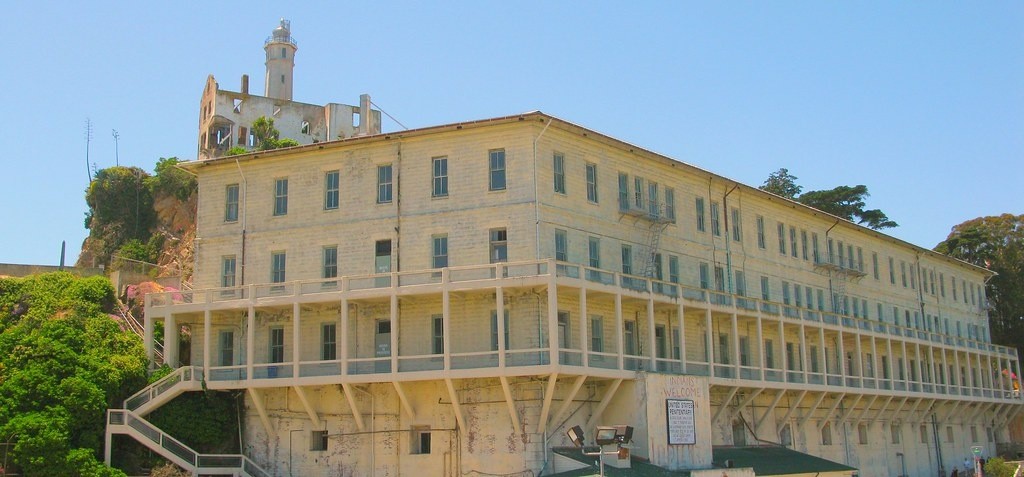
951 466 959 477
979 456 991 468
963 458 970 477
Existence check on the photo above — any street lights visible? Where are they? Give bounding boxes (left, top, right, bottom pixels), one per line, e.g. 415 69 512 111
567 424 634 477
